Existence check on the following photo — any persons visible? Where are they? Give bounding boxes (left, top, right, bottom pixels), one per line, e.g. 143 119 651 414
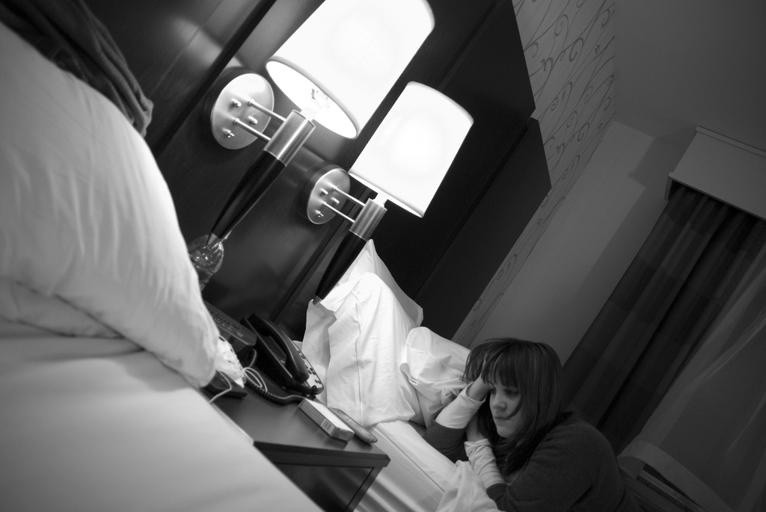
422 338 645 511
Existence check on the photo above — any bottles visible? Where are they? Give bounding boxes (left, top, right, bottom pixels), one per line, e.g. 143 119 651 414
188 230 232 291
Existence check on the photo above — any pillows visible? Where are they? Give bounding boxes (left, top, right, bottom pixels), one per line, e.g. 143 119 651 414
1 280 124 343
0 25 225 392
301 234 488 434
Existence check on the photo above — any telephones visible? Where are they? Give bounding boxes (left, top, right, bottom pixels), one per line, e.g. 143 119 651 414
240 310 324 403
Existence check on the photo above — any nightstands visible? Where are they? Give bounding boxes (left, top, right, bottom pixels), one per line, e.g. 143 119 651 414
209 373 388 512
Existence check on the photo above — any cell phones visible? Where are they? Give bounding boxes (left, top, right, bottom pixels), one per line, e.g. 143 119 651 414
328 407 378 445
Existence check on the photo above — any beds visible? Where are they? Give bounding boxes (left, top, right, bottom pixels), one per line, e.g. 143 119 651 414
273 0 555 512
0 2 337 512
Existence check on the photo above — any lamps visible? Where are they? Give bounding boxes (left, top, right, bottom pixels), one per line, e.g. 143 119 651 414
190 0 437 296
298 81 474 307
